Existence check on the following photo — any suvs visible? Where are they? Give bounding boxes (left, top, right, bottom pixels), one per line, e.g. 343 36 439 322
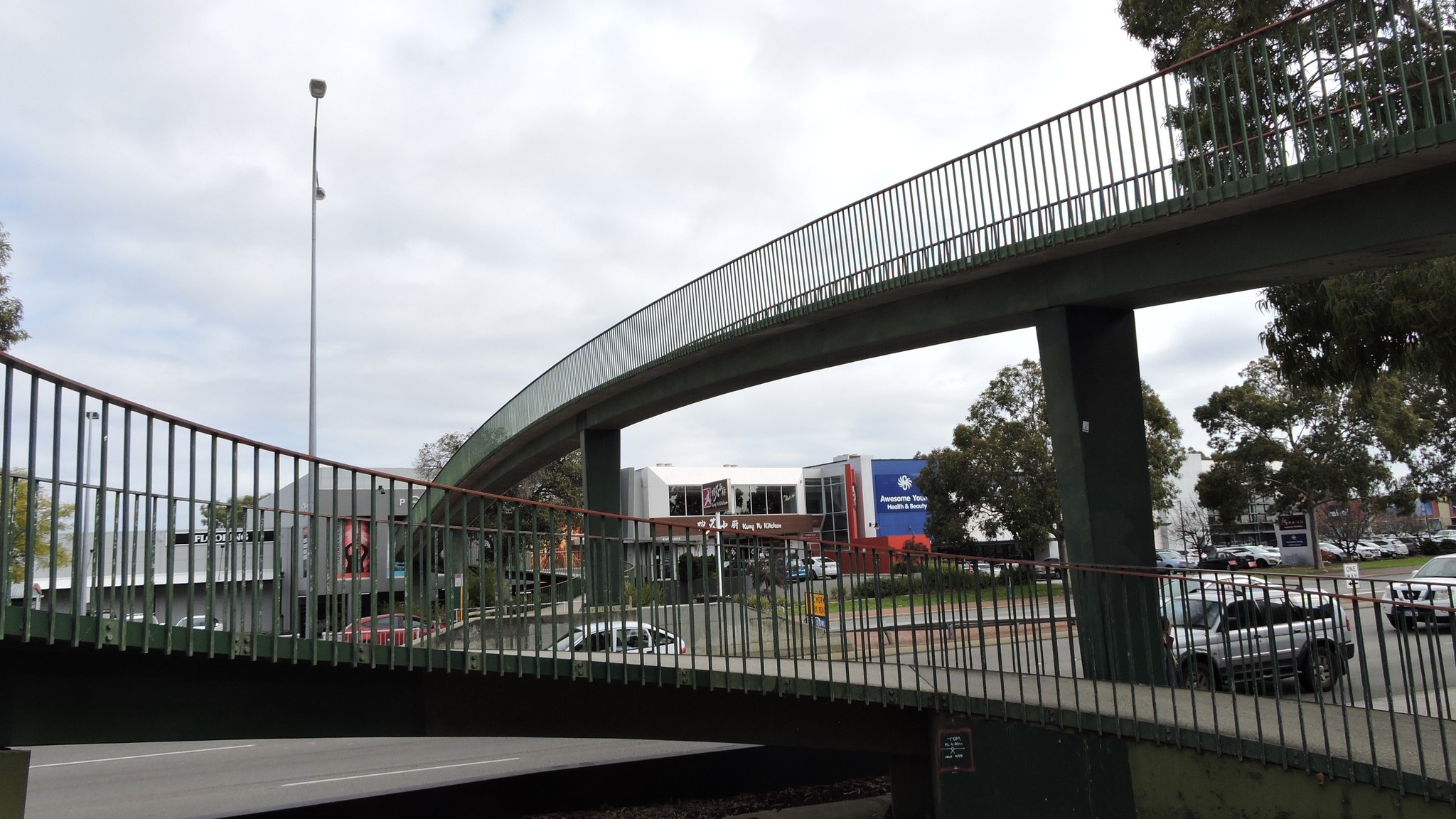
1160 583 1354 694
1380 553 1456 631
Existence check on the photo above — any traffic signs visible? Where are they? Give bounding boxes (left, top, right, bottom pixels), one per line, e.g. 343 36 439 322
1343 563 1360 589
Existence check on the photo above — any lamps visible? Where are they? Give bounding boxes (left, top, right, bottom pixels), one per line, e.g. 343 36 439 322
378 485 386 495
868 522 882 530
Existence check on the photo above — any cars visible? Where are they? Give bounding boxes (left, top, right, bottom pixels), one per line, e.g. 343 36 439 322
1156 543 1282 576
338 613 446 646
175 614 223 631
1159 573 1282 608
956 556 1062 580
724 556 840 586
126 612 165 625
87 609 117 619
547 620 686 655
1319 530 1456 563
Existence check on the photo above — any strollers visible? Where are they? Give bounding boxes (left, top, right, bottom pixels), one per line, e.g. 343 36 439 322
1320 549 1333 566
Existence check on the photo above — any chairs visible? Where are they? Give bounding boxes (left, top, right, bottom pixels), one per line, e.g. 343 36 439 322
593 632 622 653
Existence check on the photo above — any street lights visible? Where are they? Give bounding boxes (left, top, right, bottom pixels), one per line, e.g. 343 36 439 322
79 411 100 615
1166 499 1197 573
308 77 328 513
